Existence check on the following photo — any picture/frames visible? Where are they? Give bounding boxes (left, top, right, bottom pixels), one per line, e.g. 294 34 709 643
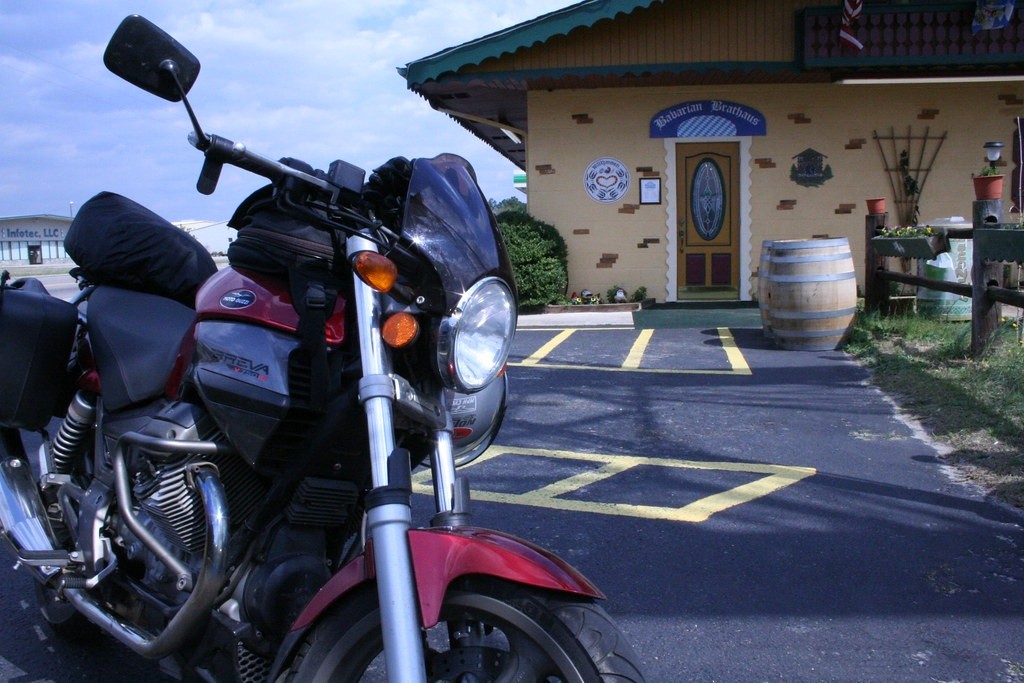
638 177 662 205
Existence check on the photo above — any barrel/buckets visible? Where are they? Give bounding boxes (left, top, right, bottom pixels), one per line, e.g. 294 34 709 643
769 235 860 351
758 235 830 341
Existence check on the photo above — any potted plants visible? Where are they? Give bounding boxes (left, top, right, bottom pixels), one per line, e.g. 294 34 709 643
969 164 1007 200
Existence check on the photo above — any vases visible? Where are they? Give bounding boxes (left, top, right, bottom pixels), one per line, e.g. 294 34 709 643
865 197 886 214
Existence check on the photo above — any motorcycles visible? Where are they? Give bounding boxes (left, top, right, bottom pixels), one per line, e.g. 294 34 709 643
0 11 654 683
